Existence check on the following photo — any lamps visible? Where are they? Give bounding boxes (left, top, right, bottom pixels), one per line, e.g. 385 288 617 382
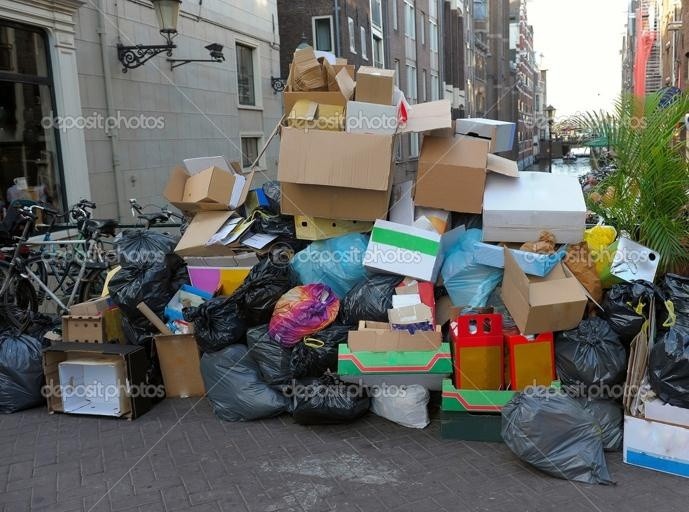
114 1 184 76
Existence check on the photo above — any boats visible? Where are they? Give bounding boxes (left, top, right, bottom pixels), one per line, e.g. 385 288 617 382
563 152 576 163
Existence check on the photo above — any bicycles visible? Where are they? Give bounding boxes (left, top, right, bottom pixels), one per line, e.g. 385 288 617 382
0 198 184 313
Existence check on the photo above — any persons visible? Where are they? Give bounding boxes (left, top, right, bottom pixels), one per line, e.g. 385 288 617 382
6 174 23 206
0 196 5 207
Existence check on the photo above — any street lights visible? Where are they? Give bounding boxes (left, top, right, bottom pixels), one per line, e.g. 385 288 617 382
543 105 556 173
